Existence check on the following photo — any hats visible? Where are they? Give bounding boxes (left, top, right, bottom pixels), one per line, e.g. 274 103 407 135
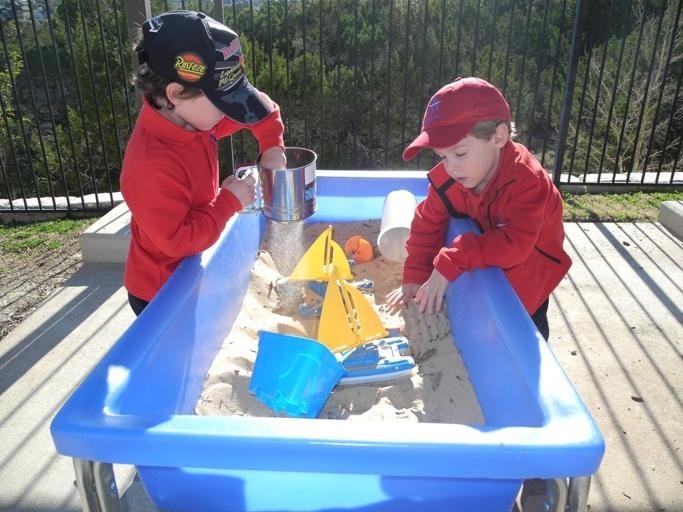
400 75 514 163
133 9 276 127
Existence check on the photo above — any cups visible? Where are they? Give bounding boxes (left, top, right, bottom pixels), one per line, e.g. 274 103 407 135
377 190 418 262
234 148 317 222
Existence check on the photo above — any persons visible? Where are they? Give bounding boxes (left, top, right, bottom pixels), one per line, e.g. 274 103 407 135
386 76 572 342
119 9 291 317
